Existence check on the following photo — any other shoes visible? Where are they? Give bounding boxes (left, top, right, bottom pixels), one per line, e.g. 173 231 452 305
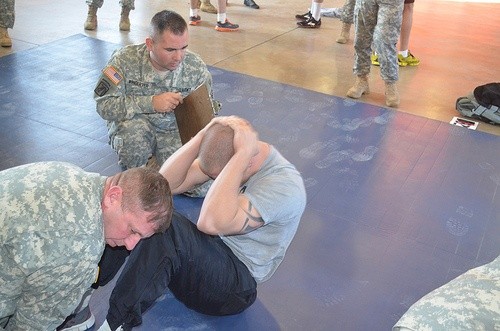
244 0 260 9
57 305 95 331
201 0 217 14
296 16 321 27
295 11 313 19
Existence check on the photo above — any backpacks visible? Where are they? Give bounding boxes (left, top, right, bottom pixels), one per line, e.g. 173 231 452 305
455 81 500 125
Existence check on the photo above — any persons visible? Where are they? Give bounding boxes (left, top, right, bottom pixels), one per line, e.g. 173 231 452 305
295 0 419 106
0 0 16 47
84 0 135 32
92 11 218 199
56 114 307 331
0 161 172 331
188 0 260 31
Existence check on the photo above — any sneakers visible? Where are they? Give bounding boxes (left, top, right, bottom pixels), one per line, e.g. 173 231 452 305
215 17 239 32
371 53 380 66
188 15 201 25
398 50 420 67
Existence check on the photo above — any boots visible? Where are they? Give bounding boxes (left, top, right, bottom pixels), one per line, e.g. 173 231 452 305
84 5 97 29
346 75 370 98
0 27 12 46
336 22 351 44
384 80 400 106
119 6 130 31
